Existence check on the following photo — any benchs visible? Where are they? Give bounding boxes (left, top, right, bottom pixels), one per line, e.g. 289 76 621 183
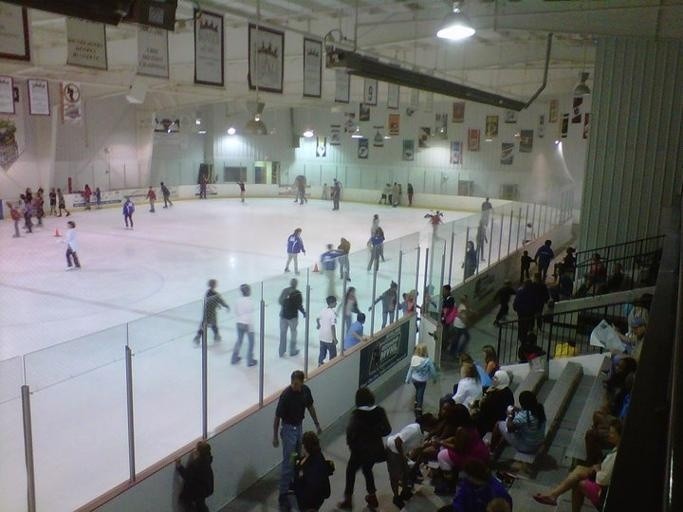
564 264 660 299
398 359 640 512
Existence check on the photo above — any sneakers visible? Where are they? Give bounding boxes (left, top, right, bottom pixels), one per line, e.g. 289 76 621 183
533 493 558 506
365 495 379 507
279 352 284 357
338 501 352 510
400 488 413 500
290 349 300 356
231 357 240 363
247 360 257 367
392 496 405 509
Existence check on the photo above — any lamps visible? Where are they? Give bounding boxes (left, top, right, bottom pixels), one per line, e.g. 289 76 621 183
350 102 364 140
239 1 270 138
434 0 475 43
572 38 592 99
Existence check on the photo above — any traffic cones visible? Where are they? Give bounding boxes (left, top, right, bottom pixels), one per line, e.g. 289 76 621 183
53 228 61 236
310 263 320 272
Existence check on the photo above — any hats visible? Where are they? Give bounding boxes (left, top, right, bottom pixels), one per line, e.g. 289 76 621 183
630 317 645 328
